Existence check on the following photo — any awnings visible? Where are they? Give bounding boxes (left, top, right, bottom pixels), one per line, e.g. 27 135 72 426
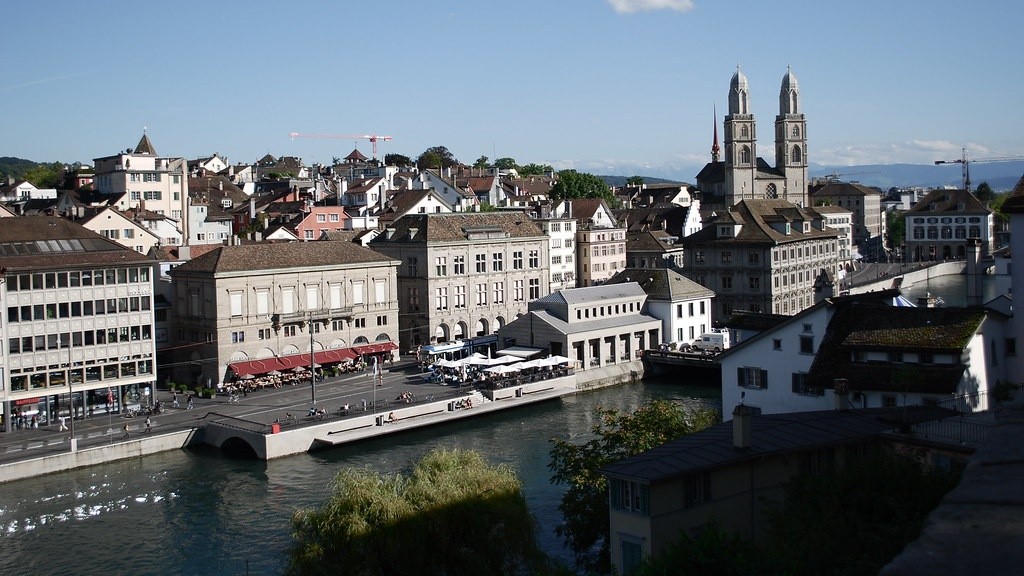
353 341 399 354
228 348 358 380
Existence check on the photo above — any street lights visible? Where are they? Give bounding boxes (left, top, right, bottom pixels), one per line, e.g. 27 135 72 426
374 355 383 386
370 356 377 414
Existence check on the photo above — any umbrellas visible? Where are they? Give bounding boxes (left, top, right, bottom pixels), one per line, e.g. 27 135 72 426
308 363 322 368
267 369 281 376
291 366 306 371
852 252 863 259
434 352 569 376
240 373 255 380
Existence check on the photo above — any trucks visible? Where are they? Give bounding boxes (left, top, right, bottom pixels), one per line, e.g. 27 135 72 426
691 332 730 354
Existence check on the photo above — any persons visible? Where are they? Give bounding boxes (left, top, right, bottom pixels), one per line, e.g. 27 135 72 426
173 393 179 407
389 412 398 423
123 422 130 439
461 396 473 410
243 383 247 397
308 404 328 419
14 408 43 429
60 416 68 431
395 391 413 407
145 416 150 432
187 395 193 409
344 403 350 416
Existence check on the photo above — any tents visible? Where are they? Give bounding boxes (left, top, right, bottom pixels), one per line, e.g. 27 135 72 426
496 346 544 358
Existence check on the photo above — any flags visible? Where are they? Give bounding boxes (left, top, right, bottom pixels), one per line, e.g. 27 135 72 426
108 387 114 405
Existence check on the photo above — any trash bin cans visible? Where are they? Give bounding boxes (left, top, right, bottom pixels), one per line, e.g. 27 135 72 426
379 415 384 426
519 387 523 396
452 401 456 411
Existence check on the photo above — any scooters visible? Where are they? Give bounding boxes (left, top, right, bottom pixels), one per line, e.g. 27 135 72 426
228 393 241 406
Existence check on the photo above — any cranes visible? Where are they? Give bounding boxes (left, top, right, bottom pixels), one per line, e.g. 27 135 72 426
825 167 882 179
934 148 1023 191
287 132 392 159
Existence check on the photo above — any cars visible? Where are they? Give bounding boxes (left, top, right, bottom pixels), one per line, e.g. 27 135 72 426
711 326 729 333
